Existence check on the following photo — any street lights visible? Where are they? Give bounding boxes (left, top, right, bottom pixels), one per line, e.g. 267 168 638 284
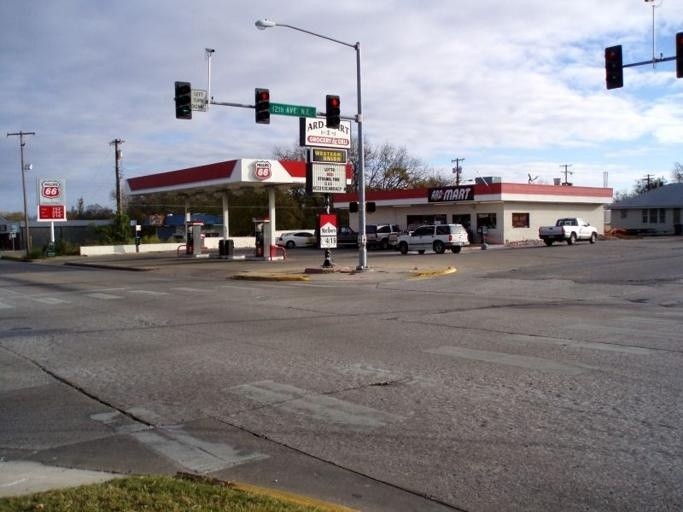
109 138 126 218
255 19 368 270
6 131 36 259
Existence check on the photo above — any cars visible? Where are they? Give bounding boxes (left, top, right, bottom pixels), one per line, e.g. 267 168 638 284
275 224 470 254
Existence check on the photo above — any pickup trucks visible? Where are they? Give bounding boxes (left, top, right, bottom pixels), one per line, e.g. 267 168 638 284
539 217 598 245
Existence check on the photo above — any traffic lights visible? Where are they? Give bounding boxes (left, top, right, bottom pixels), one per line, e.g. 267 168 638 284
175 82 192 119
605 45 623 89
255 88 270 124
676 32 683 78
326 95 340 128
